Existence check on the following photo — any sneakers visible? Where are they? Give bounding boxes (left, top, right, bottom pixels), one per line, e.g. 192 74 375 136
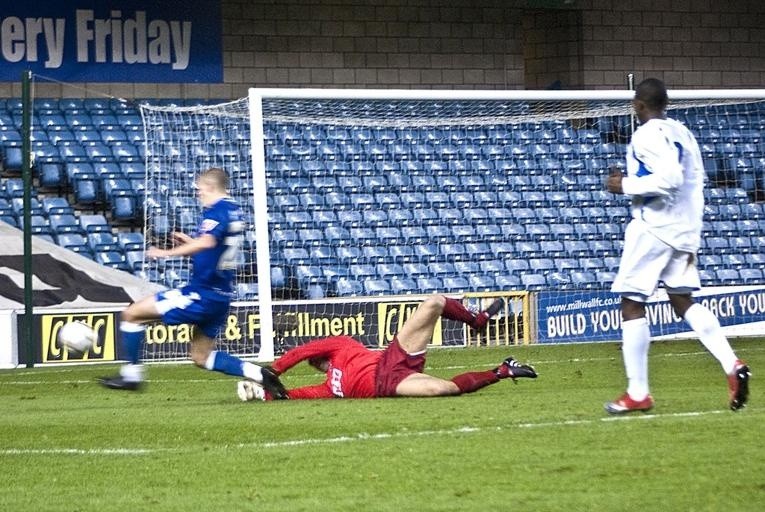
261 367 289 399
504 356 536 378
475 298 505 329
727 360 750 410
101 377 138 389
604 393 654 413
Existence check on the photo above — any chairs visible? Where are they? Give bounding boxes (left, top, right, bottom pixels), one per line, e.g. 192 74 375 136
0 94 765 301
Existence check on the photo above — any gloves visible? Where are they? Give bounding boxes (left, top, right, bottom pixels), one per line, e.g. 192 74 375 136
238 380 265 401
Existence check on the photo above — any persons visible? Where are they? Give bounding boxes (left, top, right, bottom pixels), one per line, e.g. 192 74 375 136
93 166 285 398
237 294 537 399
601 77 752 414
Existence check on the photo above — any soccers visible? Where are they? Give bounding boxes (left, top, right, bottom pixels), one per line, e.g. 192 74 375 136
59 321 90 353
